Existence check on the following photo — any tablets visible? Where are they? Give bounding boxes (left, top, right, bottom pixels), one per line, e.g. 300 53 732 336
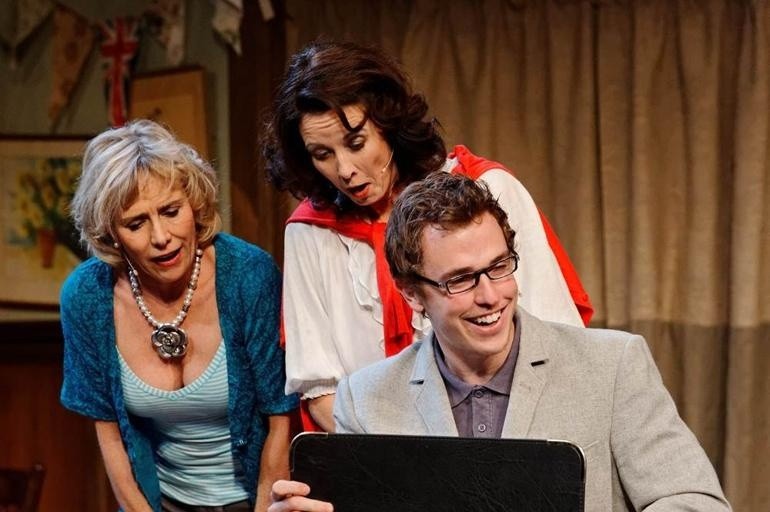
286 431 588 512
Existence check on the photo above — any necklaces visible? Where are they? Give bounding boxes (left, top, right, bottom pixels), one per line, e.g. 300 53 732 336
128 247 203 361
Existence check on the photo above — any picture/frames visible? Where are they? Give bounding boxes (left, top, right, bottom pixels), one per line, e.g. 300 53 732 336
0 131 98 313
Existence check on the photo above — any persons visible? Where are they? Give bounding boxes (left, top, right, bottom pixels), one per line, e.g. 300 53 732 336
262 42 594 437
265 170 735 511
60 118 298 510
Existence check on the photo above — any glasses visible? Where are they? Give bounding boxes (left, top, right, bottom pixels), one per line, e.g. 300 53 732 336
415 251 519 295
